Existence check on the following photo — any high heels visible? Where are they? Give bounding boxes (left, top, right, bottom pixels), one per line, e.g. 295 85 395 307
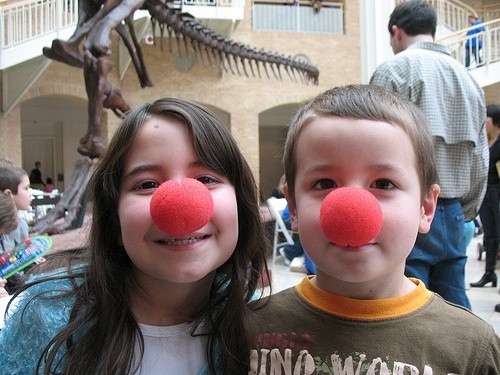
470 272 497 287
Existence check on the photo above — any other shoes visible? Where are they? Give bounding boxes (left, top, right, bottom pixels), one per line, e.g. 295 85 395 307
495 303 500 312
477 243 485 260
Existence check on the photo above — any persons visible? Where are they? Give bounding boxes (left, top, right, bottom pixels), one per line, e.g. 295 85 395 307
0 99 275 375
469 104 500 288
45 178 54 192
272 174 317 276
369 0 491 315
0 158 47 302
28 161 46 191
240 82 499 375
464 14 487 70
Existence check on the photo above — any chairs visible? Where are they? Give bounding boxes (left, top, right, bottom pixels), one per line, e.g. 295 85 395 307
267 197 298 264
